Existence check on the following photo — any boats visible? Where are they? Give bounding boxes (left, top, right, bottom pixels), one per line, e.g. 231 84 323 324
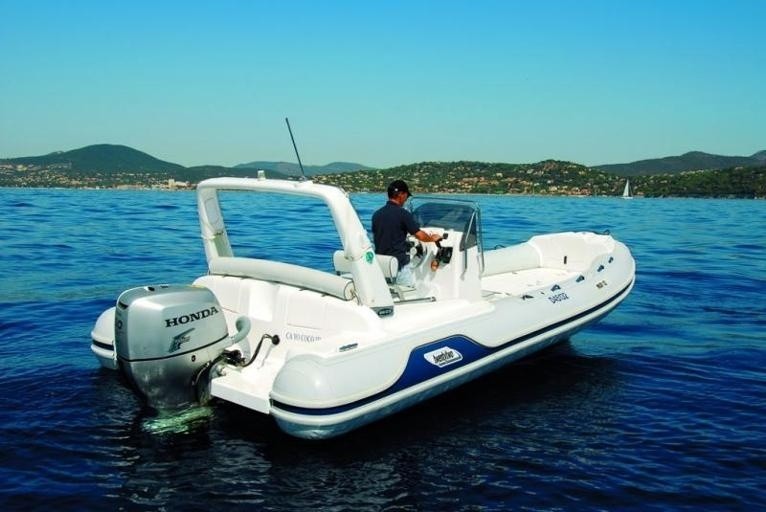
90 116 637 439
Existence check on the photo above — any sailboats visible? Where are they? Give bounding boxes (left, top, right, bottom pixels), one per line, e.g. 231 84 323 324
619 178 635 200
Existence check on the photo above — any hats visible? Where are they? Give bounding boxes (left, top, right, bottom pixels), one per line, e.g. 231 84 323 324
388 180 412 196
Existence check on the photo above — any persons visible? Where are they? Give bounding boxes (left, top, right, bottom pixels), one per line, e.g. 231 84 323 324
370 181 444 273
